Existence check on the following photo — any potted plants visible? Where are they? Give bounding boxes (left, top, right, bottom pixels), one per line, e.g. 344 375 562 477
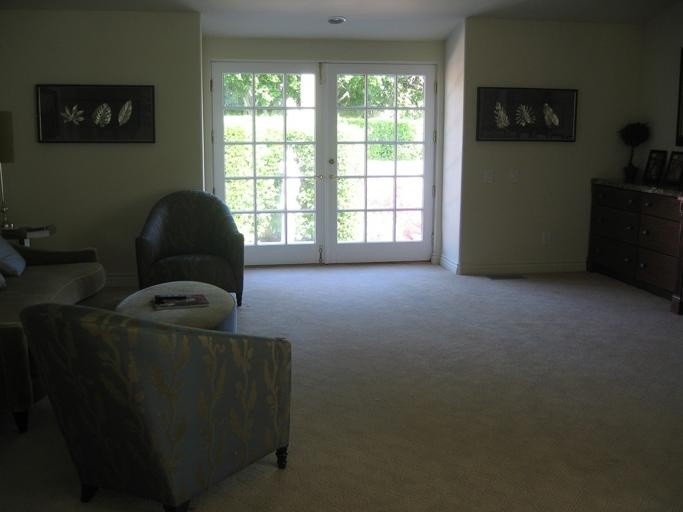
615 120 652 179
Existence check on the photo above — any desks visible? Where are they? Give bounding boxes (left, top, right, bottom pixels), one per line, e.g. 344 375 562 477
0 224 58 248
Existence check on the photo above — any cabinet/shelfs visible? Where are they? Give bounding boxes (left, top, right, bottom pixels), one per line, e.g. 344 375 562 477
586 177 683 315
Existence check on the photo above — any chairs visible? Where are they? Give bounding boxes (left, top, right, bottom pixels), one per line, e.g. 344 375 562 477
135 190 244 306
18 303 292 511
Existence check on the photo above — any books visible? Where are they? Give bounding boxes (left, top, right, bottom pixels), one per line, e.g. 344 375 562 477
152 293 209 311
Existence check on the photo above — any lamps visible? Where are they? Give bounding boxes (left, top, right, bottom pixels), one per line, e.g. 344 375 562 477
0 110 16 229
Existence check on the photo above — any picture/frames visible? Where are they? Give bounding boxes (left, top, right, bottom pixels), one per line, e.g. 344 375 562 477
643 149 667 183
665 151 683 184
476 86 578 143
675 47 683 146
35 84 156 143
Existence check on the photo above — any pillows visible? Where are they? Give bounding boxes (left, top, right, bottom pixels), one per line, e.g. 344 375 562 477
0 272 7 289
0 235 26 277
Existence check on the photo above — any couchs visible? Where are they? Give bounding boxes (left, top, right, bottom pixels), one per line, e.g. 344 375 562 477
0 244 107 434
